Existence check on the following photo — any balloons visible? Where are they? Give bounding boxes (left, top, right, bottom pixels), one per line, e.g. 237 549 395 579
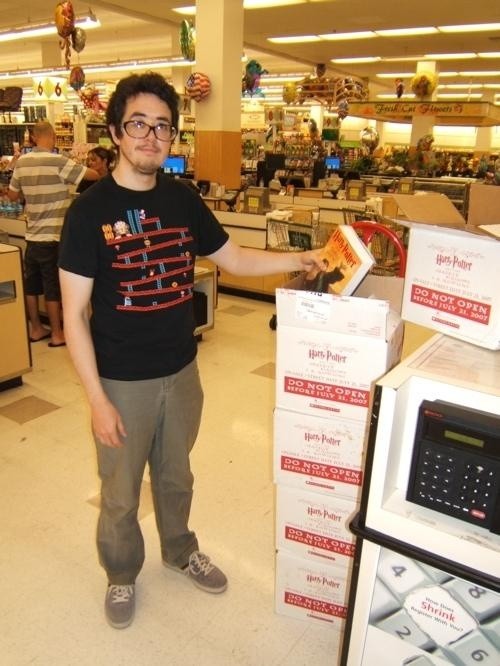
55 0 438 156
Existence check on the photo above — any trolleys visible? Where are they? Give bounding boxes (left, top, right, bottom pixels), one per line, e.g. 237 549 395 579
262 205 405 334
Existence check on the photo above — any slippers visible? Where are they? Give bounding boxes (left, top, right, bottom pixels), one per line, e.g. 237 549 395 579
30 332 50 343
47 342 66 347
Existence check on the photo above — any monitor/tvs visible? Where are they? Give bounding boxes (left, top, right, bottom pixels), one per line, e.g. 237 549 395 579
159 155 186 176
324 157 341 171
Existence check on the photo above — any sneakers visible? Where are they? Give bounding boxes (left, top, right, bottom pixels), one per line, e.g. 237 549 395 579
161 549 229 596
104 582 136 631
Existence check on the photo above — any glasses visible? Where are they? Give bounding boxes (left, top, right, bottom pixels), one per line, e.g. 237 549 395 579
120 119 180 142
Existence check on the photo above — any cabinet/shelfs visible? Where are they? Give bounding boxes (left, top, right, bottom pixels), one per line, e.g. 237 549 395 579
359 172 500 224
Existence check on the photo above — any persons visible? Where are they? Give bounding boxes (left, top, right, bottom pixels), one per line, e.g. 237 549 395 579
462 154 473 177
307 258 345 294
75 147 116 194
7 121 100 347
57 73 325 629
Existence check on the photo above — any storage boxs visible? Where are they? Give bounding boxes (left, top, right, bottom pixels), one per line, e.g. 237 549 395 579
382 192 500 350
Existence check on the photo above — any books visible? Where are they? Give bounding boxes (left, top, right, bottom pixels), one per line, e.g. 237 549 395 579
305 224 376 296
